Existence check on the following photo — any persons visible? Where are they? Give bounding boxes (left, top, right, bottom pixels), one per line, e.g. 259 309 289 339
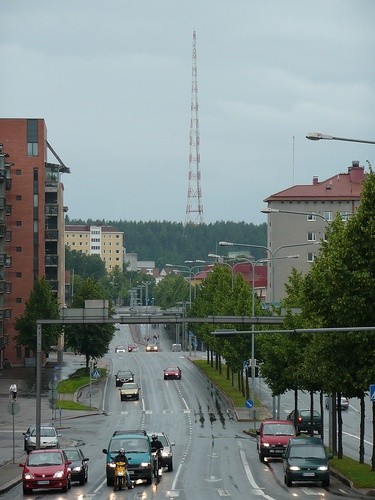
150 433 164 452
10 382 17 400
114 448 133 489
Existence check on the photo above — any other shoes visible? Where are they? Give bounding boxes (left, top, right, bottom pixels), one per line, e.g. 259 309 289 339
128 487 133 489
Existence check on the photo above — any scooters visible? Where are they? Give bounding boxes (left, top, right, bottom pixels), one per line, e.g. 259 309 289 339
152 448 163 482
111 461 127 491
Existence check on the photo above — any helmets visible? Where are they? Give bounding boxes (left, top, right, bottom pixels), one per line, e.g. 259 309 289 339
152 434 158 442
119 448 125 455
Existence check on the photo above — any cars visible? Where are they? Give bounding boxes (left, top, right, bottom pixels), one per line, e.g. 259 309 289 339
145 430 175 472
115 343 158 353
284 436 330 486
103 429 153 486
18 449 72 494
23 425 61 452
118 383 142 400
55 447 89 485
163 366 181 380
287 408 323 436
326 393 349 410
255 421 296 462
115 369 135 387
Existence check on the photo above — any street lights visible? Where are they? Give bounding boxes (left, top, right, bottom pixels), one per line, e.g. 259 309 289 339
158 258 273 393
208 253 301 398
260 206 367 454
219 239 326 418
306 131 374 145
